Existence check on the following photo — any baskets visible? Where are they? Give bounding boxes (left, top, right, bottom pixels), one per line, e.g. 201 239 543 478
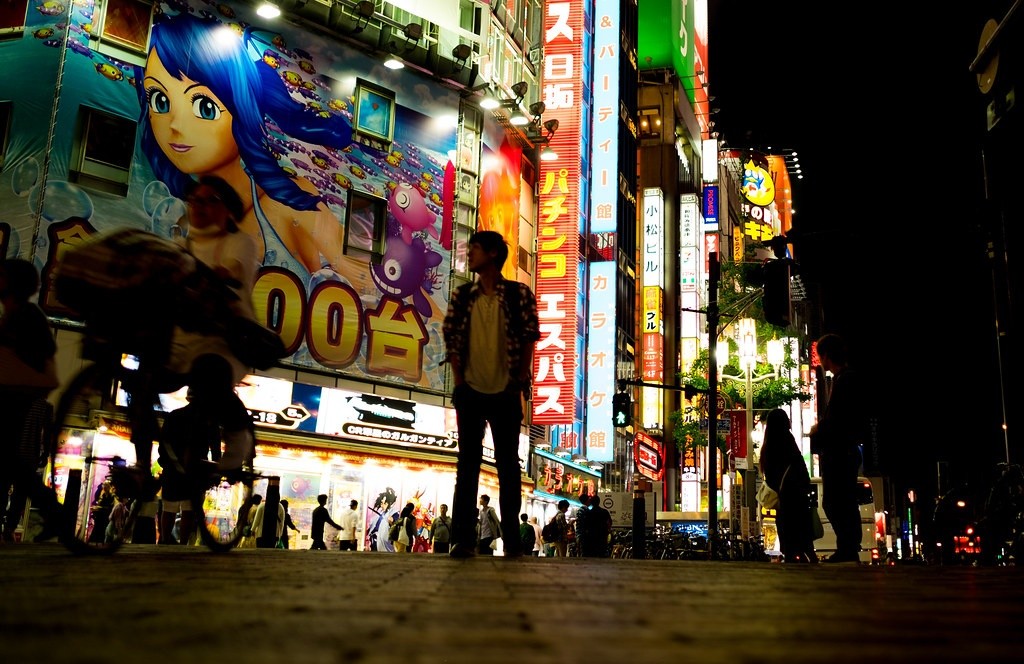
81 307 175 369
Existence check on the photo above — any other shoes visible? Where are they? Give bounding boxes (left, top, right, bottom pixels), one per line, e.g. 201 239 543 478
449 543 476 557
821 550 859 563
218 428 254 471
33 503 63 542
113 464 160 497
504 550 523 561
3 528 16 545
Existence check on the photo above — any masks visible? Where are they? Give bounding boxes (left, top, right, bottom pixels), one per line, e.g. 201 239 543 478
187 223 220 235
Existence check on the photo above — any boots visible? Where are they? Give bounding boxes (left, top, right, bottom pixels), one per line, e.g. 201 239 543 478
161 511 176 544
180 510 196 546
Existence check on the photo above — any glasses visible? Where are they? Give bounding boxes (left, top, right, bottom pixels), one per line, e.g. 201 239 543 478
187 194 220 209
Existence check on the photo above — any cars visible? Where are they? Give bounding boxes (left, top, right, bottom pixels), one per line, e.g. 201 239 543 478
876 525 1024 570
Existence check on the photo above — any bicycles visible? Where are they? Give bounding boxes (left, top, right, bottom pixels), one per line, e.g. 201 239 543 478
564 520 772 562
27 305 266 558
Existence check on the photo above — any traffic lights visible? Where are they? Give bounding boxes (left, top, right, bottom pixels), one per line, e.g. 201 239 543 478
612 392 631 427
761 257 792 328
810 340 826 370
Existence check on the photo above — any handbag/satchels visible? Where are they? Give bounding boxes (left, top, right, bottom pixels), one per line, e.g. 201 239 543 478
754 480 780 509
0 346 58 396
277 539 285 549
810 507 824 539
488 507 503 537
223 315 287 371
243 526 251 536
398 517 410 546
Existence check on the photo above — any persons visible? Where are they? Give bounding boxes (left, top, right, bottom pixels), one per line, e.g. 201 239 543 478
761 409 821 562
309 493 418 553
239 495 300 549
521 495 613 557
0 176 261 543
811 334 866 562
428 494 502 556
443 231 539 556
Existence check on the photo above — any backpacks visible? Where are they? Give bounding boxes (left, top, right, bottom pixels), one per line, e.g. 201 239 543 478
542 512 562 543
524 525 535 544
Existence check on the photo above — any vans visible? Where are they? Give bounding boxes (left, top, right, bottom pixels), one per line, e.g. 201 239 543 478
808 476 882 567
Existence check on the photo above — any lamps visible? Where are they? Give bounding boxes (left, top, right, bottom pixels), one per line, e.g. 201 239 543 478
256 0 280 18
509 110 528 126
351 1 375 29
685 83 710 90
645 56 654 71
690 96 716 103
544 119 560 141
511 82 529 105
402 23 423 53
384 54 405 68
529 101 545 124
679 70 705 78
695 108 720 115
452 45 471 71
533 438 604 471
773 152 803 214
69 415 108 448
480 94 499 109
540 142 558 160
